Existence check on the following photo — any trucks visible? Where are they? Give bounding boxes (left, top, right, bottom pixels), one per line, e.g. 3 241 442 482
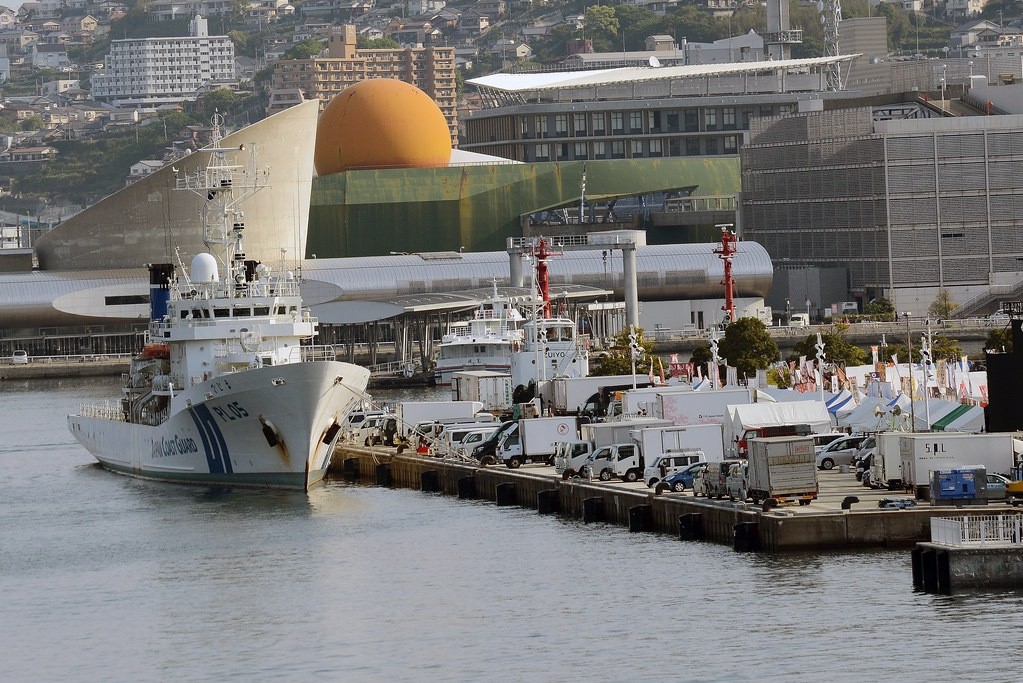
850 430 973 493
734 423 814 459
726 436 820 507
346 401 725 482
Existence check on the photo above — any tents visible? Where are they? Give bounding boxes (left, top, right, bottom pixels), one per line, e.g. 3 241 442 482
694 378 833 402
826 387 985 432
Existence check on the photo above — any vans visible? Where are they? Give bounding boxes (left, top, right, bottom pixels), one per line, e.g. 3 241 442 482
9 350 28 365
702 460 740 500
805 433 849 448
644 447 707 488
816 436 873 469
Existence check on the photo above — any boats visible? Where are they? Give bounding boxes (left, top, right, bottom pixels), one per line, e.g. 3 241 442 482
66 107 371 494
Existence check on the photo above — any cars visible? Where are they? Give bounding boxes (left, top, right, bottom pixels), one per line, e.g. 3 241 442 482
663 462 709 492
986 474 1013 500
692 467 708 497
